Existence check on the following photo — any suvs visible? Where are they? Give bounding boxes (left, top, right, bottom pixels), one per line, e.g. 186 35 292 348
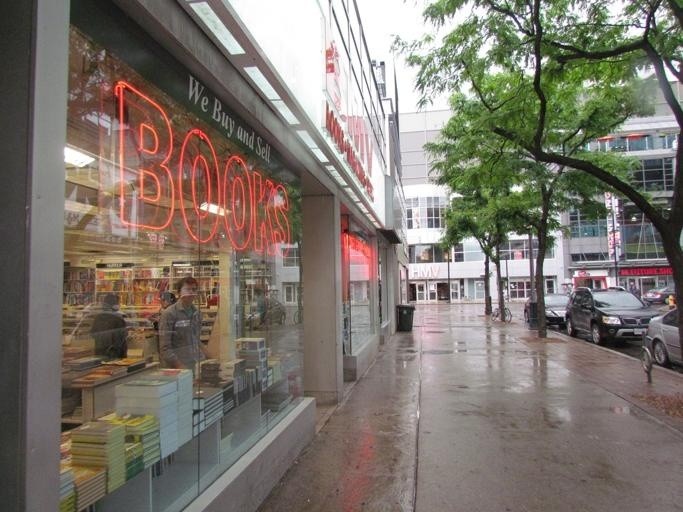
562 285 661 348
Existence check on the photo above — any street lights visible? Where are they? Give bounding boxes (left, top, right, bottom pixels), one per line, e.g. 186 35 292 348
604 205 623 286
443 248 450 305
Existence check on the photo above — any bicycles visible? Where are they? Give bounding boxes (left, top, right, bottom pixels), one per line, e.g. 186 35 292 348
489 304 513 324
293 305 303 324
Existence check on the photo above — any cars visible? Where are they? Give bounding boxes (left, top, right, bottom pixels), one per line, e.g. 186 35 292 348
642 284 674 306
522 292 573 332
642 307 682 370
243 296 288 332
61 301 154 361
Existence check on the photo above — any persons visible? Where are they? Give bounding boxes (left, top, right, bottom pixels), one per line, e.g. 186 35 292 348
92 295 129 358
159 275 209 370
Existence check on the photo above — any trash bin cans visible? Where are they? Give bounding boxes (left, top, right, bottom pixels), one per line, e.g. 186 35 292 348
396 304 416 331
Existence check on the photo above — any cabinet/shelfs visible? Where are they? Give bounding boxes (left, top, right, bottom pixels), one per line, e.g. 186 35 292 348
61 260 276 310
59 354 160 425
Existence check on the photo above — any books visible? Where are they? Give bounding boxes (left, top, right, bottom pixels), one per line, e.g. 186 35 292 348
61 338 294 511
62 268 220 338
